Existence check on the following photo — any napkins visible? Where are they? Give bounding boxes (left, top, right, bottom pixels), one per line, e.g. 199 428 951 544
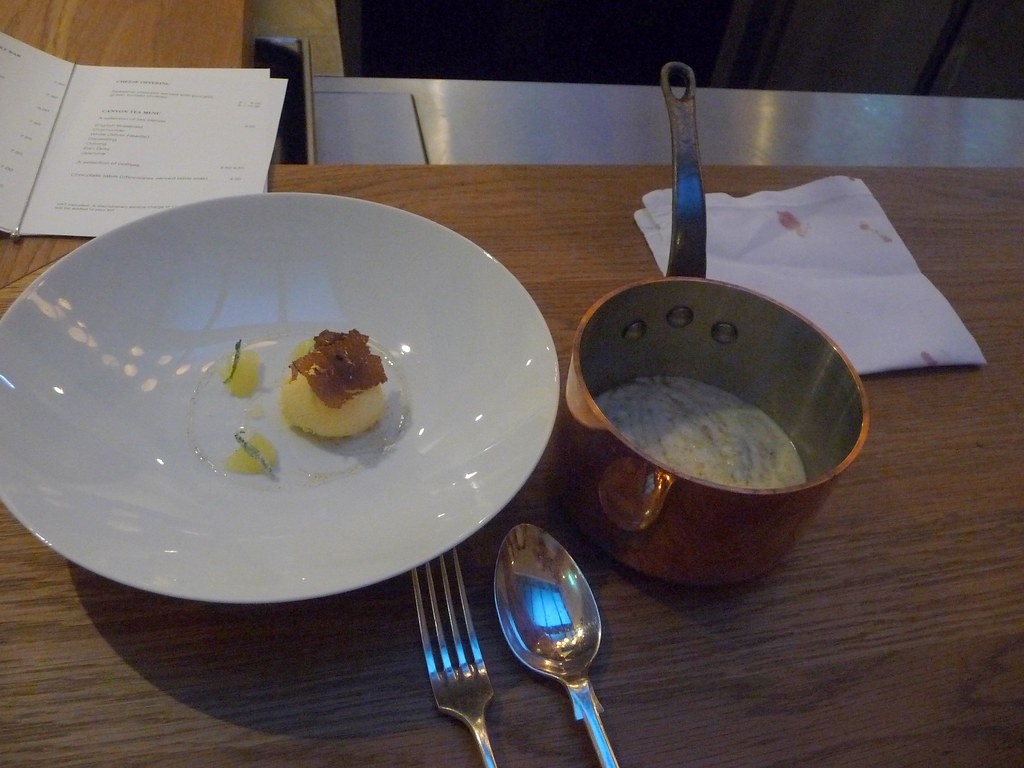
634 170 989 376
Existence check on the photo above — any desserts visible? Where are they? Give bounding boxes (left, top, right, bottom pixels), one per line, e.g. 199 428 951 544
279 328 391 440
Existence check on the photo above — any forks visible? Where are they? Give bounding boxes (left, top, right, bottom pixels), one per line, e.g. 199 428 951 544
412 547 500 767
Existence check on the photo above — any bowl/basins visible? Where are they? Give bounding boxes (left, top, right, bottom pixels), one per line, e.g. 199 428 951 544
1 193 560 603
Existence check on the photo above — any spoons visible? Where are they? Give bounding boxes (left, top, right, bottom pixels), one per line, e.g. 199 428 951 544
494 524 622 767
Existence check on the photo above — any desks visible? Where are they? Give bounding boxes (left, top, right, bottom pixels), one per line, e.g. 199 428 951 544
0 0 1024 768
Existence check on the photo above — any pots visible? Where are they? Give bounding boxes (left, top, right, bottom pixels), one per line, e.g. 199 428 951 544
560 61 870 587
